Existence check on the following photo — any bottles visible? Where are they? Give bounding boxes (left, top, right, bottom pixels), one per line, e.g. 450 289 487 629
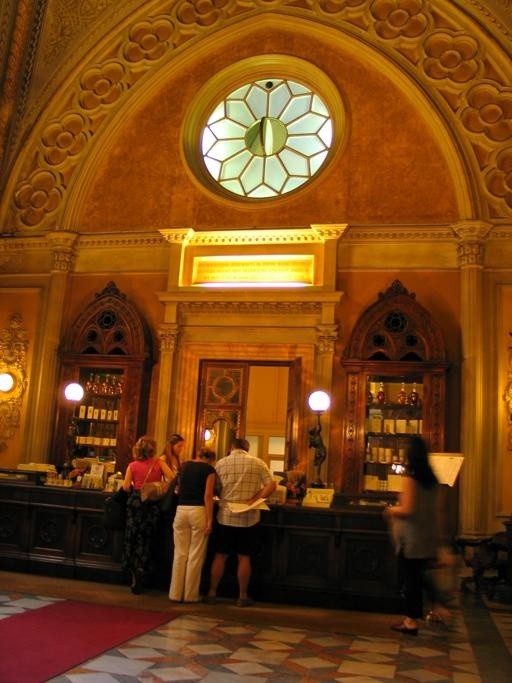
366 381 419 406
86 372 123 395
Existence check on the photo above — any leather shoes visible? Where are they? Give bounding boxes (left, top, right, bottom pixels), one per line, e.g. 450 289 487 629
389 620 418 635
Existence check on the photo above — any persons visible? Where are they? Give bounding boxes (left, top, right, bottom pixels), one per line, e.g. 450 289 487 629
124 435 174 593
160 434 185 482
384 437 440 634
164 446 219 603
207 438 276 606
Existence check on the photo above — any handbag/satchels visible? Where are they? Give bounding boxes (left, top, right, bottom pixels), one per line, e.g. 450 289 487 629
142 481 172 503
160 491 178 513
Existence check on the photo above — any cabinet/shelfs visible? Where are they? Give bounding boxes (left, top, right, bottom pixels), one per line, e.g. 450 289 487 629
51 281 155 480
338 279 453 499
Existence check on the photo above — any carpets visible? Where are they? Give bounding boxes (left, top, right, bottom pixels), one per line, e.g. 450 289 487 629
0 598 181 683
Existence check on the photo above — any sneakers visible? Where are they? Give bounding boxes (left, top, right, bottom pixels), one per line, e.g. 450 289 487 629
201 593 217 605
237 597 254 607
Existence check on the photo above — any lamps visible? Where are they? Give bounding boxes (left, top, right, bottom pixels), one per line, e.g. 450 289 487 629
64 383 84 466
307 391 331 488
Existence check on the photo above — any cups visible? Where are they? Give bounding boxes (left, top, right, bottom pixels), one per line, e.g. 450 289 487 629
77 436 116 445
